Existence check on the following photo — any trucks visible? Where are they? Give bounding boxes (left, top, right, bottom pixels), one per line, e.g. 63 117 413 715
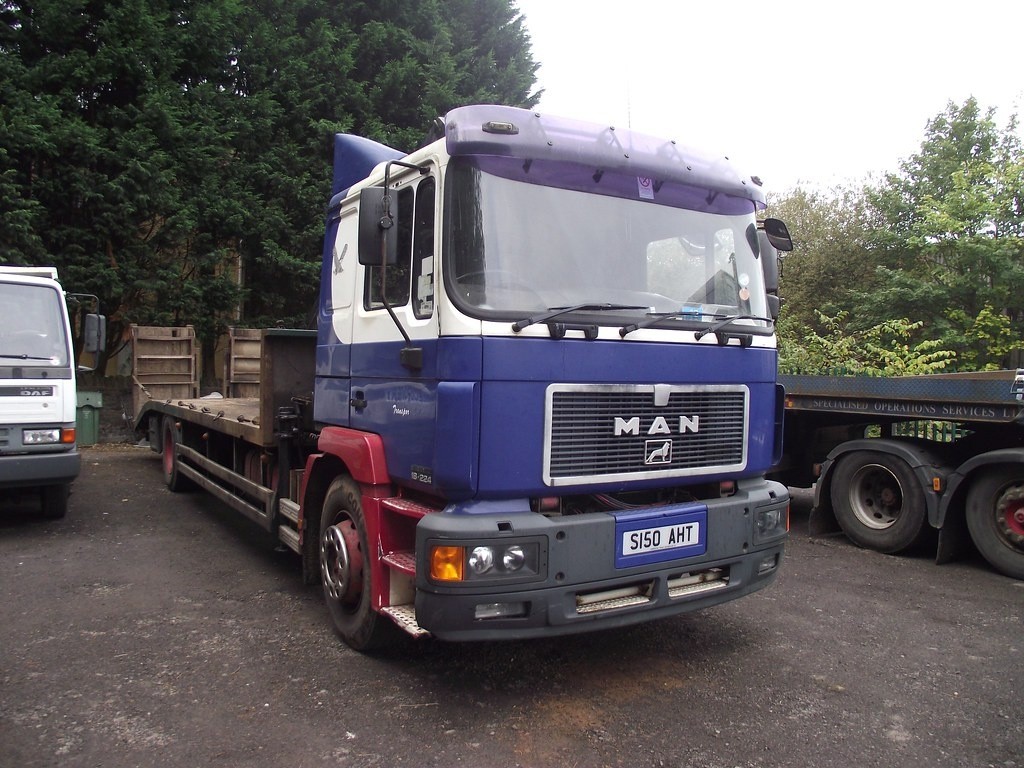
778 372 1023 583
132 103 793 654
0 265 108 518
